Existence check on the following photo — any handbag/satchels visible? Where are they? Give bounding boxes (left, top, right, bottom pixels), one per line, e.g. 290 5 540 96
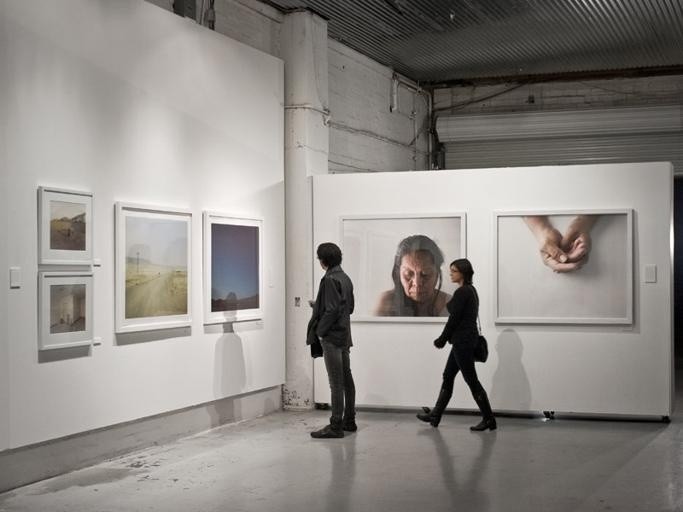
473 335 488 363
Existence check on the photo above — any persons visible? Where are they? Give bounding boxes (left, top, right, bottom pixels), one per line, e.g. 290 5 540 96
521 215 603 274
416 258 497 431
306 242 356 438
373 235 453 316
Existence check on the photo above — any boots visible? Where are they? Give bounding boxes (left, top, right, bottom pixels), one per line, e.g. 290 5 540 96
415 390 451 427
470 391 496 432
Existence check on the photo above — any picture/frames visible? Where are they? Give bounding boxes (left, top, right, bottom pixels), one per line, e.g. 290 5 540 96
113 200 193 335
337 212 467 323
202 210 264 326
37 185 94 266
37 270 94 351
492 206 635 326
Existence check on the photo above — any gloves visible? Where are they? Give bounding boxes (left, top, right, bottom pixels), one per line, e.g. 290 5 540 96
433 336 445 348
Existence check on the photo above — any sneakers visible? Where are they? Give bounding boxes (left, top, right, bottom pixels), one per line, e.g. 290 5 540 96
342 424 356 432
311 429 344 438
